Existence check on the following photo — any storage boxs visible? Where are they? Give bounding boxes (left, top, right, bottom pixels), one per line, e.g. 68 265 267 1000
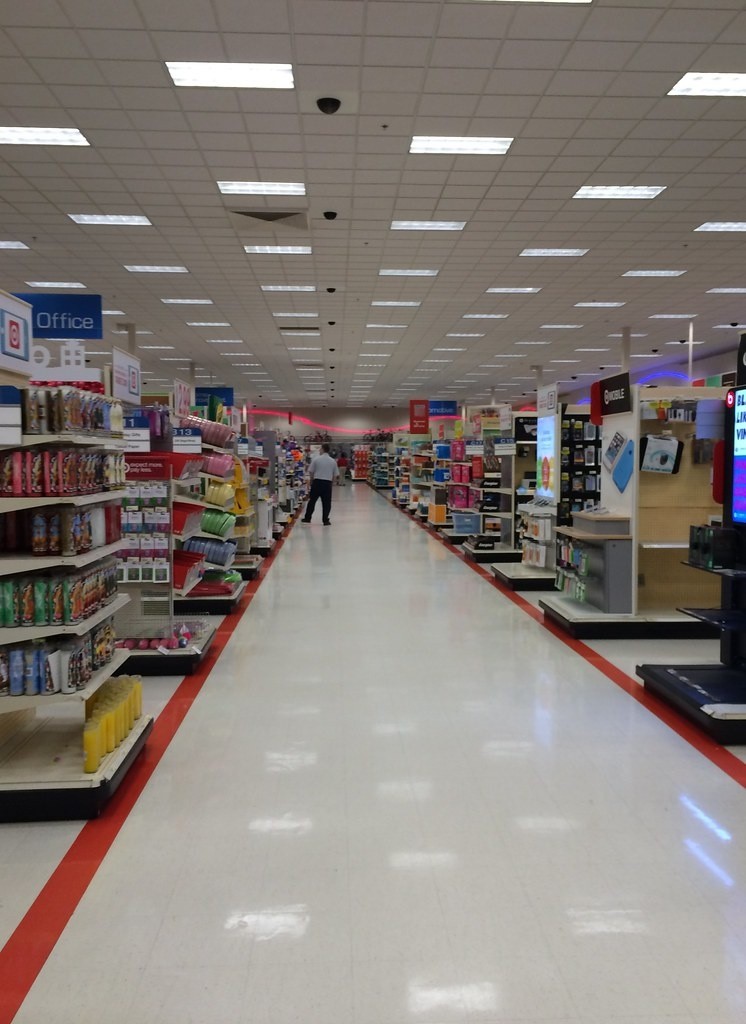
399 484 409 492
452 516 478 531
436 446 451 460
453 466 462 484
468 536 495 550
413 456 429 463
428 503 446 524
400 456 411 464
473 500 500 512
447 486 480 508
461 466 470 483
418 501 428 514
433 468 450 482
451 441 465 460
483 473 502 488
430 486 446 505
484 517 501 536
472 456 483 487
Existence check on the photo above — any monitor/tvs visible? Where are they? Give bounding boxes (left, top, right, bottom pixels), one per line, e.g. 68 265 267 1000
721 387 746 531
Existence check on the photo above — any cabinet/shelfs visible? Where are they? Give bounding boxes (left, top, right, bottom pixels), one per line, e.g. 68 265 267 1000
491 372 746 746
0 290 310 824
462 439 538 563
367 452 453 532
439 441 501 546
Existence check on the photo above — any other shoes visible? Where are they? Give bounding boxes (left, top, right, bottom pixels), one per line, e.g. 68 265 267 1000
301 518 311 522
324 522 331 525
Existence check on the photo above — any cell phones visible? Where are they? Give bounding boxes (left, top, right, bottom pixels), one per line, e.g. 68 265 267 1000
602 431 634 494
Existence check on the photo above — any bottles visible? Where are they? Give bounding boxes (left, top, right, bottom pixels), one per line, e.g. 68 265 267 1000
81 674 144 774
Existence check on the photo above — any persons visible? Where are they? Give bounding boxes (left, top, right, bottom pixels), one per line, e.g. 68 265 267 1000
301 444 340 525
337 451 348 486
